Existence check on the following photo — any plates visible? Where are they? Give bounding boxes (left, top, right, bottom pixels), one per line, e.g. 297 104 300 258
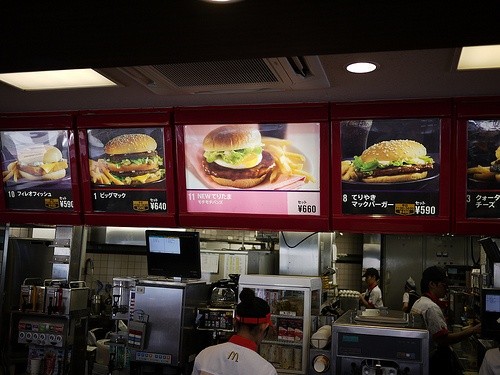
186 136 314 189
340 158 440 194
92 165 166 188
2 159 71 186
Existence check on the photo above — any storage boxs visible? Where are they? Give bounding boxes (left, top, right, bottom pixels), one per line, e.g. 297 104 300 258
8 227 28 238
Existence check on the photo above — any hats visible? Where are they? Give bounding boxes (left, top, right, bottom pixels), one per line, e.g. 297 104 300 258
420 266 454 286
407 277 416 288
361 268 379 277
234 313 274 330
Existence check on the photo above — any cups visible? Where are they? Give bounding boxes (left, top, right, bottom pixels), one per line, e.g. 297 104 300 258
313 356 329 372
31 358 42 375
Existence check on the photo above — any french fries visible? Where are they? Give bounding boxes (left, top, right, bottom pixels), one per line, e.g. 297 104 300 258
3 162 20 182
341 161 359 181
263 139 317 184
89 160 124 185
467 146 500 179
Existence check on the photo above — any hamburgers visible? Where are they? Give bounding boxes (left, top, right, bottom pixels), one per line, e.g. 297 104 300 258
203 125 275 188
17 144 67 180
353 139 433 183
105 134 161 185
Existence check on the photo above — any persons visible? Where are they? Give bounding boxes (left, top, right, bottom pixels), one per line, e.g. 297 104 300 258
409 266 482 375
480 318 500 375
359 267 383 308
191 288 278 375
402 279 420 313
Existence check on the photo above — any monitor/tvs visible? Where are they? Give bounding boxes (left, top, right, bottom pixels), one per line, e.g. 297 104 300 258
145 229 201 279
480 288 500 340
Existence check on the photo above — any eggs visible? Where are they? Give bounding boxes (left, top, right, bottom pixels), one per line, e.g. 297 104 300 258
339 290 360 298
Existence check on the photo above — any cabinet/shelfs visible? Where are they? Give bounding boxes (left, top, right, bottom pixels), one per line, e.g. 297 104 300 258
238 274 322 375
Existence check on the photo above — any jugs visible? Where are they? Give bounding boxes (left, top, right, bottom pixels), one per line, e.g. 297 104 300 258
209 278 235 306
